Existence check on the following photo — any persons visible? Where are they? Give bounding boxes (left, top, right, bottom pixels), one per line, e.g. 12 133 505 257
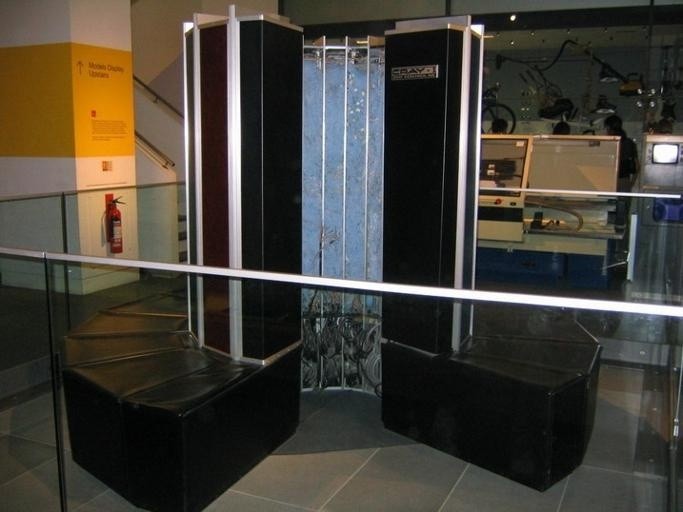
492 119 507 134
604 115 639 262
553 122 570 134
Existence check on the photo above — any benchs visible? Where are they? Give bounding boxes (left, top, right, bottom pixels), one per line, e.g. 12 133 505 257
379 282 607 494
62 288 302 512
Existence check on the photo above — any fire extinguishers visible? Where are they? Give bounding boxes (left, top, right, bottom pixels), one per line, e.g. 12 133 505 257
101 193 126 254
